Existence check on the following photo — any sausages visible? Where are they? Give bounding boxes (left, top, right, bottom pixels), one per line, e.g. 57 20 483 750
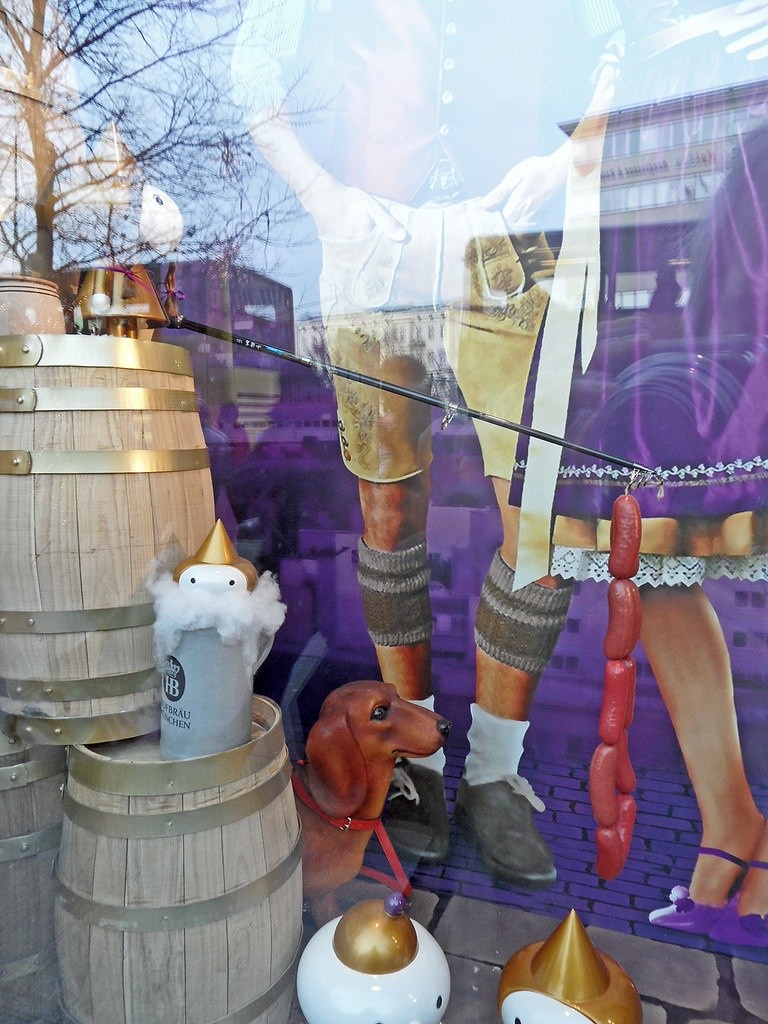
588 495 642 883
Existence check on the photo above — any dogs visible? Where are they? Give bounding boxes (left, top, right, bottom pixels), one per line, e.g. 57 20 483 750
289 679 456 933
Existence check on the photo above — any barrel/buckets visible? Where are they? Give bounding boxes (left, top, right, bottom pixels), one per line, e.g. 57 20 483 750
50 693 304 1024
0 746 67 1024
0 334 214 745
